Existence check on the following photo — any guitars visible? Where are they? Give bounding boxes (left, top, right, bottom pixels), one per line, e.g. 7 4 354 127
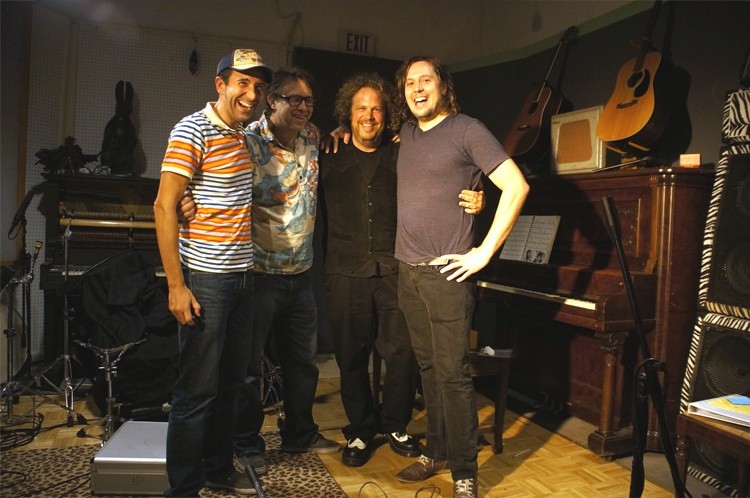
503 23 578 157
595 0 669 142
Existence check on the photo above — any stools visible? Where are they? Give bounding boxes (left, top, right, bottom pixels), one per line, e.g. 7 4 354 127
74 334 149 445
675 410 750 498
469 350 511 453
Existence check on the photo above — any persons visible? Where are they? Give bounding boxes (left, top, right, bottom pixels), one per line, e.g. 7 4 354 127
153 48 273 498
394 55 530 498
312 72 486 468
177 67 400 475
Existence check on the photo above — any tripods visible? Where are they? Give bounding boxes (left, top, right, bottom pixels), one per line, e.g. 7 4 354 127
0 209 99 427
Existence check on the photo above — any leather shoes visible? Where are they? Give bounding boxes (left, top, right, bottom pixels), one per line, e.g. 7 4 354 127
385 432 421 458
343 436 369 466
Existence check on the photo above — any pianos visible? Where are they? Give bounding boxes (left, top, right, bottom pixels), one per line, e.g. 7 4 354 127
37 170 185 389
471 162 718 464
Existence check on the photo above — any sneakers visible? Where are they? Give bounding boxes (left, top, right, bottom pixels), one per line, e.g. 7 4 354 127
453 478 478 498
204 470 266 494
238 454 266 475
281 433 341 454
395 454 451 482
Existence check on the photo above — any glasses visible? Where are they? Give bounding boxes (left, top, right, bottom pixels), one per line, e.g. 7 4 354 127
277 94 316 107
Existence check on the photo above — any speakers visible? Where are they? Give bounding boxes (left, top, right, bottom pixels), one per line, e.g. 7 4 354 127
698 142 750 319
674 310 750 498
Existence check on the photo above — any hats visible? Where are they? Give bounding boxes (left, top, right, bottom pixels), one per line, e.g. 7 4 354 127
217 49 273 84
